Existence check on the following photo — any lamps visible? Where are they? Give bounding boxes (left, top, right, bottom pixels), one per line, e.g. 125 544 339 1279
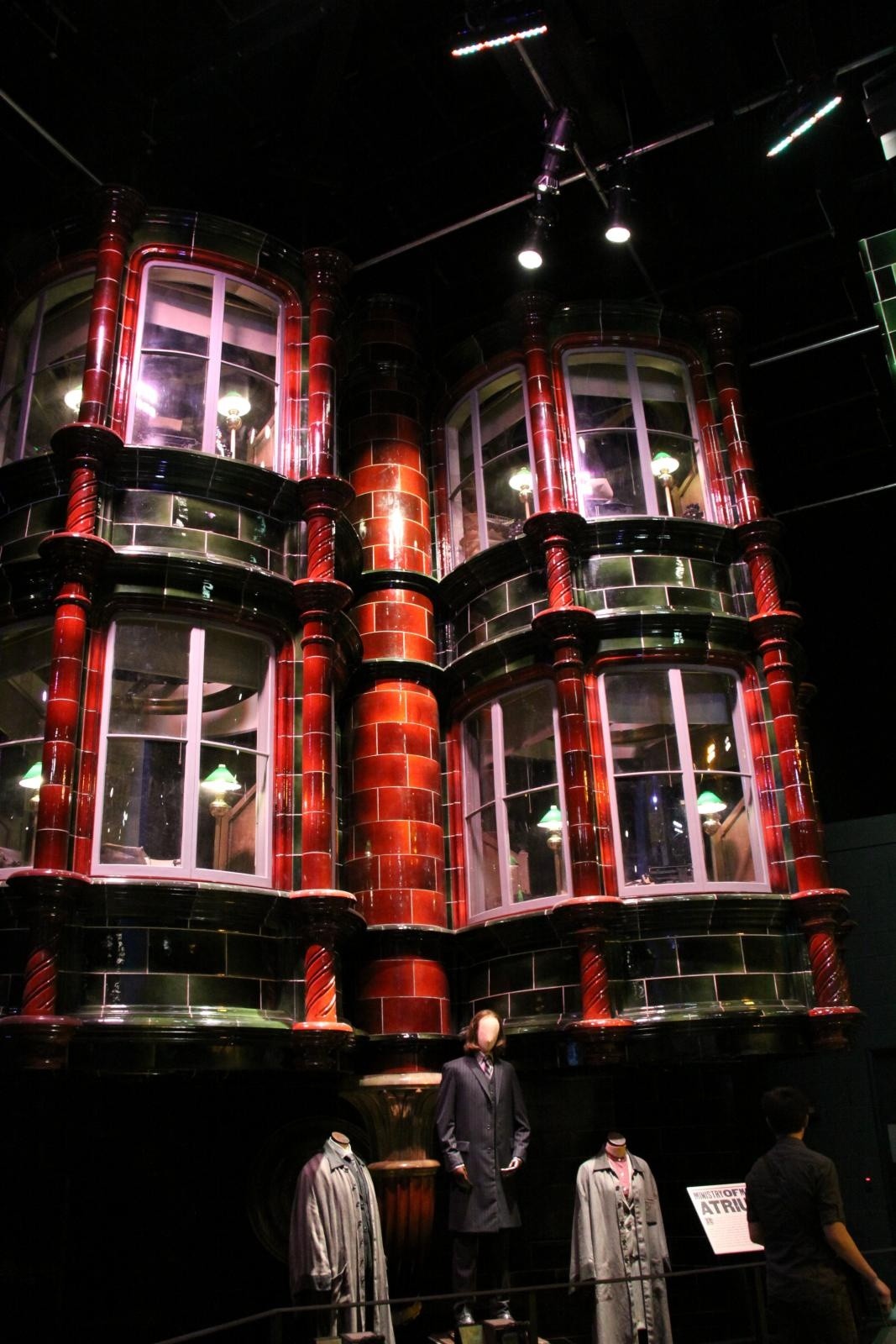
602 185 637 244
651 452 682 517
537 804 570 892
697 791 727 882
534 108 578 193
510 856 520 867
64 384 83 413
508 467 538 519
218 391 251 460
202 763 243 869
19 761 42 865
516 213 556 270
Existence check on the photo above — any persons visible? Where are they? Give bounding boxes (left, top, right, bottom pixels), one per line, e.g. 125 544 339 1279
287 1130 395 1344
745 1085 891 1344
432 1011 530 1326
567 1133 673 1344
